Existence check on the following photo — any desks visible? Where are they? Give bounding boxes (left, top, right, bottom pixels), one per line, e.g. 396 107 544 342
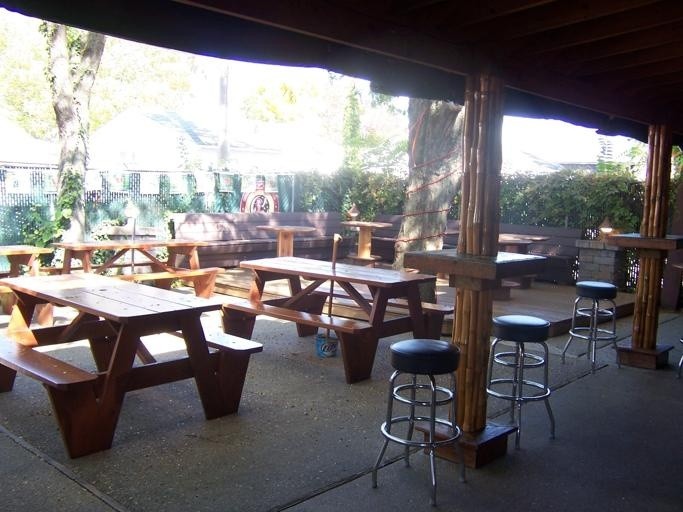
239 257 437 385
0 273 222 457
0 238 209 315
341 221 393 267
499 233 550 288
256 226 316 257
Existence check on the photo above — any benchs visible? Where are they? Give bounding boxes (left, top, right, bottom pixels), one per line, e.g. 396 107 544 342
209 285 455 385
171 212 360 270
498 223 581 284
371 214 459 261
0 322 264 459
1 260 225 315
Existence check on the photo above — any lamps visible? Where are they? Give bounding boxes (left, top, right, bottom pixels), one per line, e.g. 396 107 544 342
600 215 612 233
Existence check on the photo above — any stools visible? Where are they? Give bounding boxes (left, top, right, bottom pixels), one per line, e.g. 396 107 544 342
560 281 621 375
486 315 556 448
372 339 467 508
